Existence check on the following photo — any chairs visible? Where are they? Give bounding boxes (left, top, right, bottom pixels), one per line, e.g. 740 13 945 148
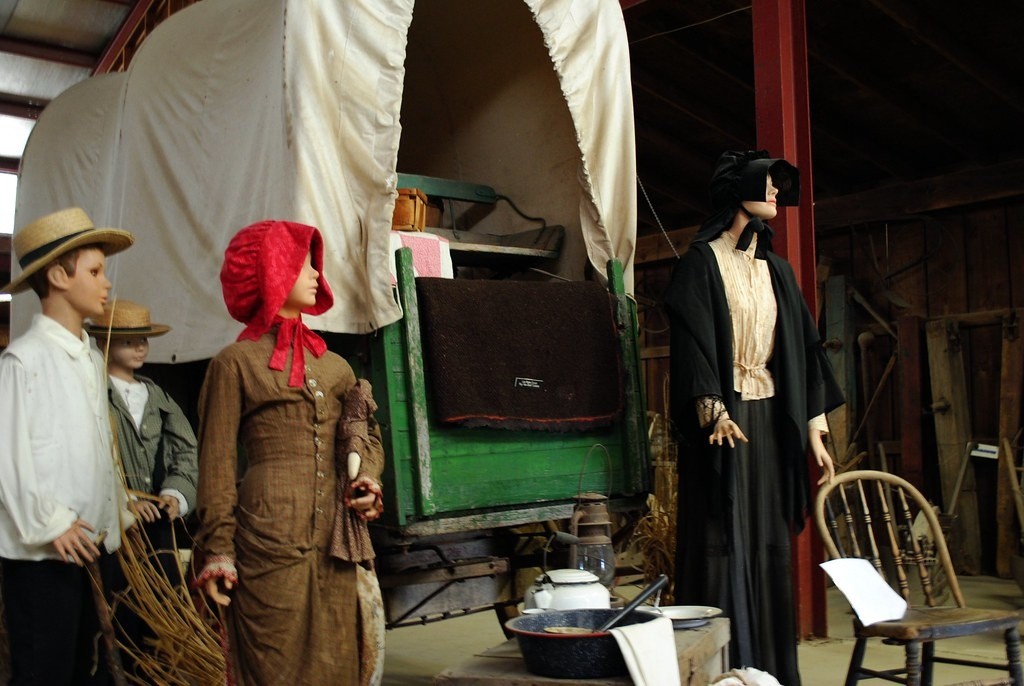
817 469 1024 685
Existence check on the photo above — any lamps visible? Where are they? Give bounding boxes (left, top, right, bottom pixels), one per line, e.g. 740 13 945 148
567 444 617 601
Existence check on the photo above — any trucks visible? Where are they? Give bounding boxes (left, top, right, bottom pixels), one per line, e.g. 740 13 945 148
0 0 657 631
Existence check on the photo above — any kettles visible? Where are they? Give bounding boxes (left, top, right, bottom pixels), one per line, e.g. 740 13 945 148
525 570 610 610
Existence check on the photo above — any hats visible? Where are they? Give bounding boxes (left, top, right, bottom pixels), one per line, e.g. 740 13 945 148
83 299 171 337
688 149 801 260
220 220 334 388
0 207 135 294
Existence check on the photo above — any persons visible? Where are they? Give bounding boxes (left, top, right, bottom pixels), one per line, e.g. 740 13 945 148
0 204 133 686
192 218 386 686
666 146 848 686
83 302 197 686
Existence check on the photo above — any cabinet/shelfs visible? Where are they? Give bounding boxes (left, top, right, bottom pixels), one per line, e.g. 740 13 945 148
430 617 732 686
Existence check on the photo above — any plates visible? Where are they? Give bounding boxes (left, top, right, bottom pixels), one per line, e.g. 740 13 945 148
637 605 723 628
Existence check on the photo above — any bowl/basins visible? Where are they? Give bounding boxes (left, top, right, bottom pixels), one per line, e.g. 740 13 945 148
507 609 662 676
